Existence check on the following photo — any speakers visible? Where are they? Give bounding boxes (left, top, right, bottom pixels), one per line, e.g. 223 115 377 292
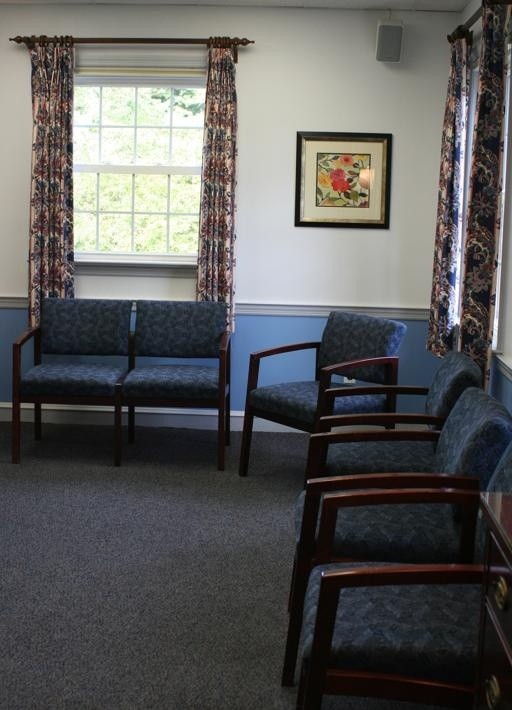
375 19 405 64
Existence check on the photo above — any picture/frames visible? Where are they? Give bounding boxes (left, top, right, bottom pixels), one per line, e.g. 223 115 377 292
288 129 393 232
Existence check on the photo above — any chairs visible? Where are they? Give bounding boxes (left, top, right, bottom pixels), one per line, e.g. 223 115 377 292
278 437 511 710
285 386 512 616
9 294 135 469
239 307 411 477
295 346 485 464
127 296 231 471
